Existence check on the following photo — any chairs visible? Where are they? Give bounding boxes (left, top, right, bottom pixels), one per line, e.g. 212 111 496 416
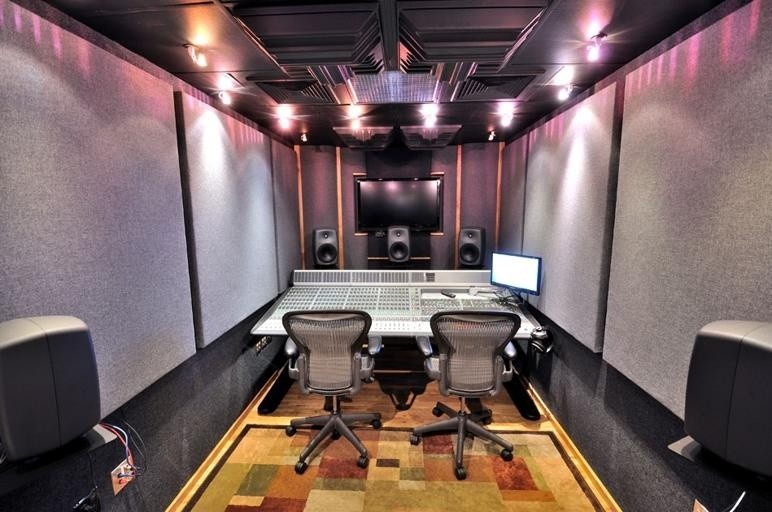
410 309 521 479
282 309 382 475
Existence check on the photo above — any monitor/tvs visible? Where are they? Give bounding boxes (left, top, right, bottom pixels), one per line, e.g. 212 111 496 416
489 251 543 306
354 175 443 234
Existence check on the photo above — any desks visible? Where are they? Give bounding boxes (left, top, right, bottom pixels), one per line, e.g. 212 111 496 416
0 424 146 512
250 269 548 420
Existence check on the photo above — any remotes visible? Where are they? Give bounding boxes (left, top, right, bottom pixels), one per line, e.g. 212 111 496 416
441 287 457 298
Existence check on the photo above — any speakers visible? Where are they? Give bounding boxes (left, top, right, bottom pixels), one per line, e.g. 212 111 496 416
313 228 338 268
458 227 485 267
0 315 102 462
386 225 411 264
682 318 771 480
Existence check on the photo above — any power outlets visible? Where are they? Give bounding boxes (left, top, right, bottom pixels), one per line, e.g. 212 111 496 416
110 454 136 496
